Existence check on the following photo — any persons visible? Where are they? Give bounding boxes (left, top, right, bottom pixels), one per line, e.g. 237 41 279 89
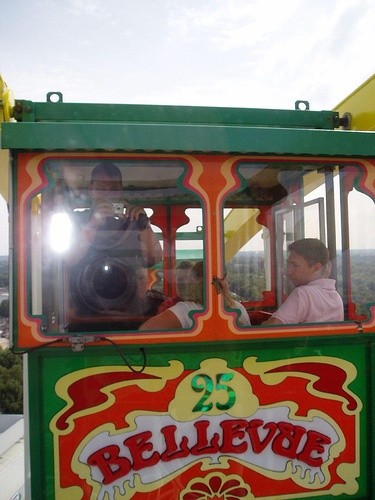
157 260 194 314
138 261 252 333
260 239 344 326
64 162 162 332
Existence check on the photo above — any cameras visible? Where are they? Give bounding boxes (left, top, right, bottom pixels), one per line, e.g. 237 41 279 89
110 202 124 214
